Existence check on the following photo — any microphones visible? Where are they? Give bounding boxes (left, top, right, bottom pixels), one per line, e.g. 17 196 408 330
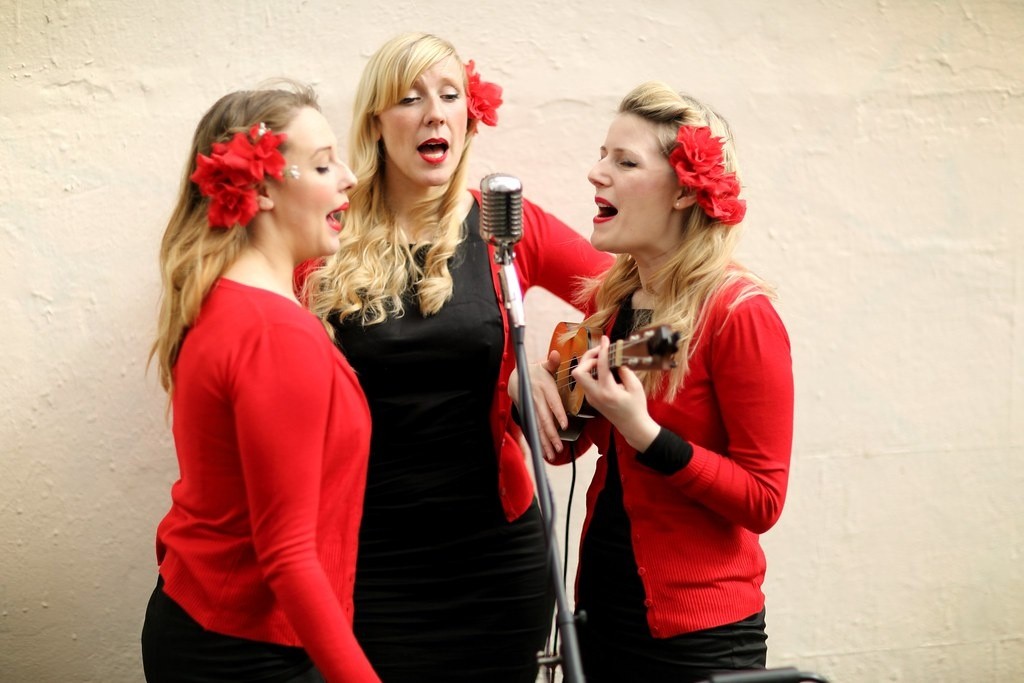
480 173 525 346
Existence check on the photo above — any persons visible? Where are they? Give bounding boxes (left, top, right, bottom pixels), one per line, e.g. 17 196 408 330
141 77 380 683
291 33 617 683
508 81 793 682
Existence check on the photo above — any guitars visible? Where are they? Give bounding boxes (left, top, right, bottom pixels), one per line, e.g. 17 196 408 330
546 321 683 442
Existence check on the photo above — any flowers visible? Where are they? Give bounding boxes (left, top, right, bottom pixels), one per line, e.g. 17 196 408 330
186 121 301 231
667 125 749 227
464 57 503 127
